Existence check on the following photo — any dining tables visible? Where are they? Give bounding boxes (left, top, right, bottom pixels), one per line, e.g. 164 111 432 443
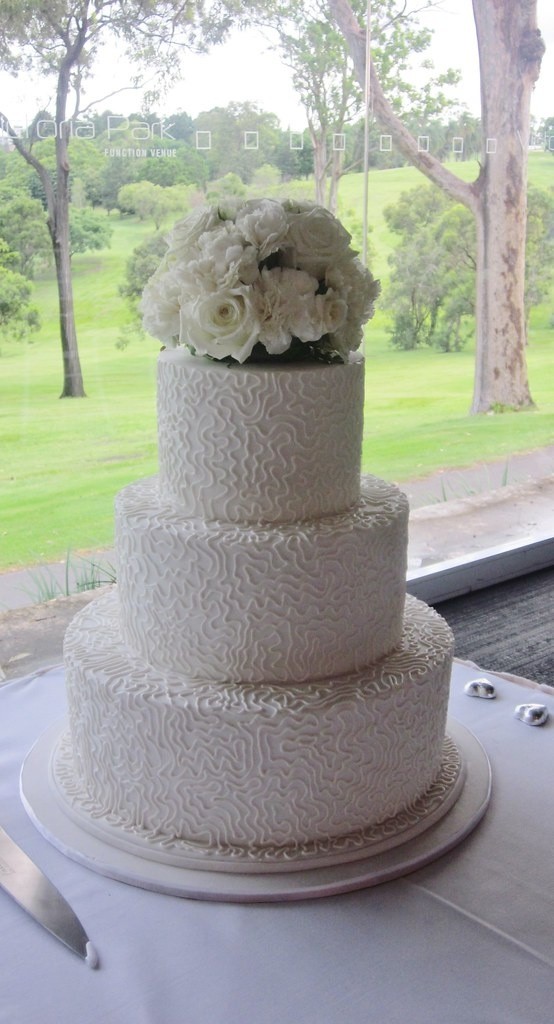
0 656 554 1024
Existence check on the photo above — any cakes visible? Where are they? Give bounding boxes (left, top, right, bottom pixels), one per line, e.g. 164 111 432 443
58 196 458 860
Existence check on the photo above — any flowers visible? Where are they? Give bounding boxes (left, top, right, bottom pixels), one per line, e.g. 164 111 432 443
135 178 384 364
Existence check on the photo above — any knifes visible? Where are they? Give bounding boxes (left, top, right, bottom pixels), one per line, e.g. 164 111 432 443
0 827 100 966
